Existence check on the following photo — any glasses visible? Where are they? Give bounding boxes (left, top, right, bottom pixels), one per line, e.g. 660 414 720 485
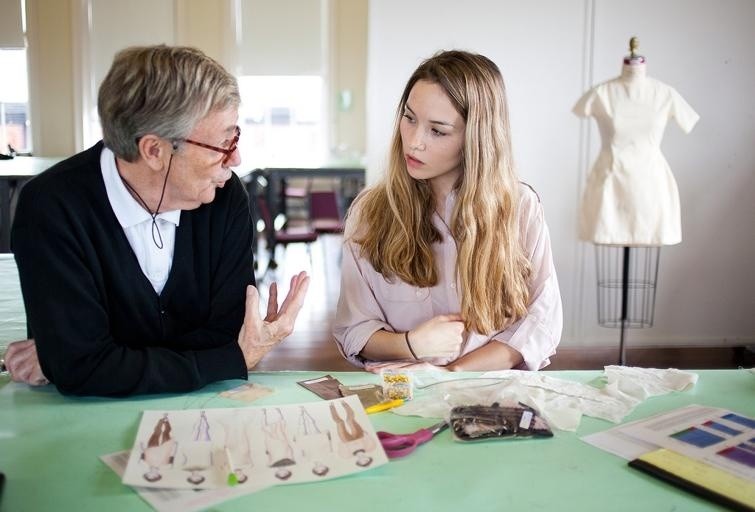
134 126 241 165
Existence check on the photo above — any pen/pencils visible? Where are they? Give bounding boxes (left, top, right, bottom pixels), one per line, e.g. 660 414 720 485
365 398 407 414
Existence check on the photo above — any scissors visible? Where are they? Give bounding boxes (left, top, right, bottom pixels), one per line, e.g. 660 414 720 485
376 420 449 457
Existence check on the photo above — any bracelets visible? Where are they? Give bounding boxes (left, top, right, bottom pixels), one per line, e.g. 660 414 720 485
405 330 420 361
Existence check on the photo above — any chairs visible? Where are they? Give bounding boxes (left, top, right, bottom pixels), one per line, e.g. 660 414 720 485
257 192 345 281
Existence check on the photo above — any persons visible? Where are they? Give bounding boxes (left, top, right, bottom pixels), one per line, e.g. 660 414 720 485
571 55 700 247
3 44 310 399
333 50 563 374
139 399 377 485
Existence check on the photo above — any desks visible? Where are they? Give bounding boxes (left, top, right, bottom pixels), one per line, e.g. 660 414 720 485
0 366 753 511
